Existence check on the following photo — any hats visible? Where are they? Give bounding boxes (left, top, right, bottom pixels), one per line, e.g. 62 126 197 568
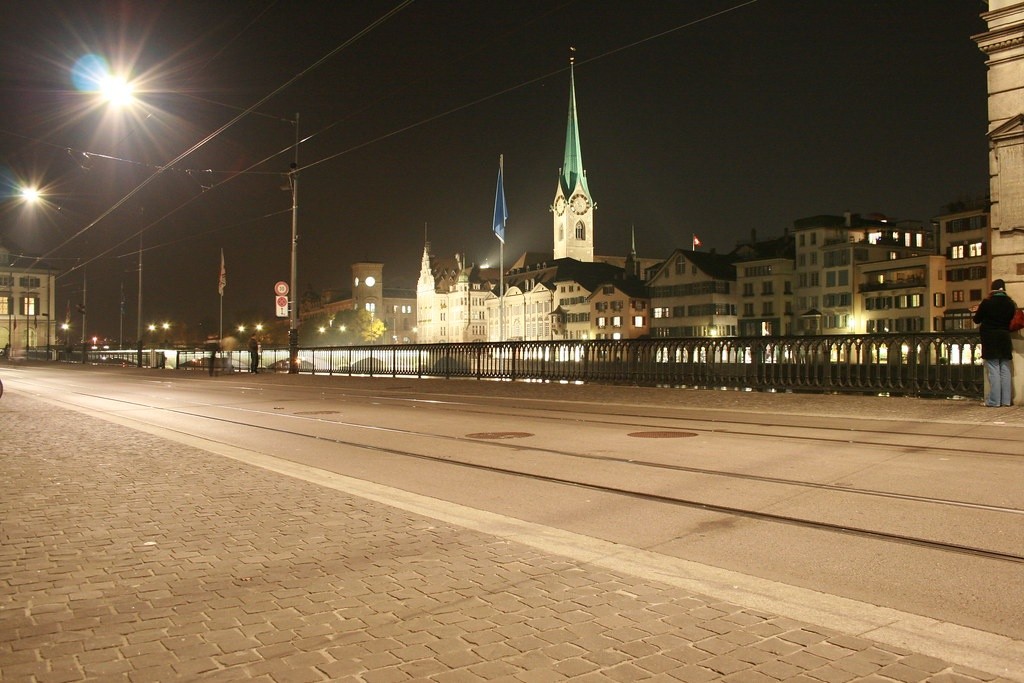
991 279 1005 291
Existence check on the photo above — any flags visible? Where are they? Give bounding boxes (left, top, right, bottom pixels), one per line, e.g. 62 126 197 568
120 283 127 314
693 233 703 247
34 312 37 329
14 318 16 330
493 154 508 244
65 299 71 324
218 248 226 296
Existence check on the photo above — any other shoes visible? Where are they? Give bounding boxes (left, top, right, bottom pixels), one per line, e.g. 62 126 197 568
979 402 1000 407
1000 401 1010 407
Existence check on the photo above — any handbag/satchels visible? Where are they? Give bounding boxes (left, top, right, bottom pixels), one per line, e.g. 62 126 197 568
1008 300 1024 332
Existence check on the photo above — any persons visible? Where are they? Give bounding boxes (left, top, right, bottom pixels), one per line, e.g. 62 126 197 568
973 279 1016 408
65 343 73 364
5 343 9 354
248 333 258 374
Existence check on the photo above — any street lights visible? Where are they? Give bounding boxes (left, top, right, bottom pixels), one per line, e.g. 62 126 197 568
364 277 376 377
